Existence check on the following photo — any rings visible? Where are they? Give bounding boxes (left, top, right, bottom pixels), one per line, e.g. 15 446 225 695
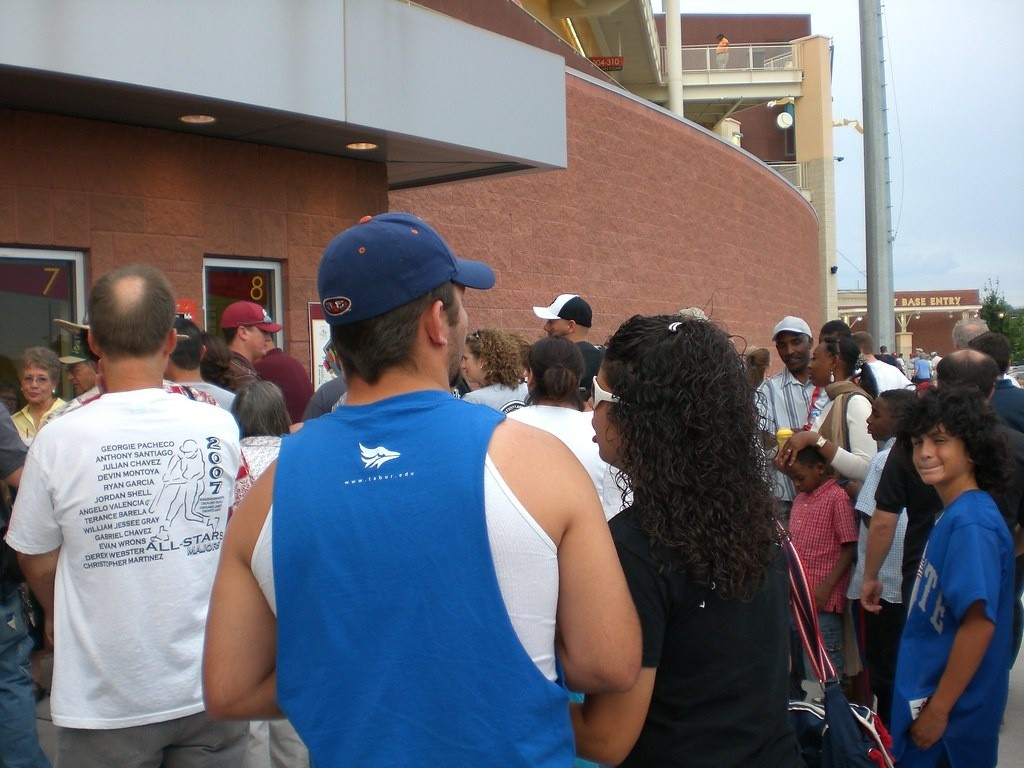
787 449 791 452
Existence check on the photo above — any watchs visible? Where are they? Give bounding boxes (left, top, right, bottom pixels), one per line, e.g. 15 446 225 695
816 436 827 449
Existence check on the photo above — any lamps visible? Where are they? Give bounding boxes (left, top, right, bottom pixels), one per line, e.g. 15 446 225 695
831 266 838 273
834 156 845 162
973 313 979 318
833 119 863 134
733 130 744 139
916 314 921 320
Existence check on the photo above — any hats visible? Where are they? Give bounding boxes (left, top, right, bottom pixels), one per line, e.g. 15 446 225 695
931 352 937 356
772 316 812 342
58 341 91 364
220 300 284 333
892 352 899 357
910 354 914 356
53 318 190 343
532 293 593 328
317 212 495 326
915 347 923 352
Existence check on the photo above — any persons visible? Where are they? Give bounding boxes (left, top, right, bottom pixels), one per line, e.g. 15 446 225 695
0 318 254 506
202 213 643 768
716 34 729 68
751 314 1023 768
161 300 349 485
0 399 53 768
449 294 634 521
5 262 252 768
568 314 804 768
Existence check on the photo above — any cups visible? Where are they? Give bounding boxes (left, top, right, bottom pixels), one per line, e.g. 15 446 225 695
776 428 794 453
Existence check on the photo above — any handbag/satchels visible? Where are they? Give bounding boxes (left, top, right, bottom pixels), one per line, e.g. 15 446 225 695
766 509 895 768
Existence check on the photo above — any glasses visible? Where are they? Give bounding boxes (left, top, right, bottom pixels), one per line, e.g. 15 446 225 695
22 375 51 384
471 330 480 341
591 376 631 410
